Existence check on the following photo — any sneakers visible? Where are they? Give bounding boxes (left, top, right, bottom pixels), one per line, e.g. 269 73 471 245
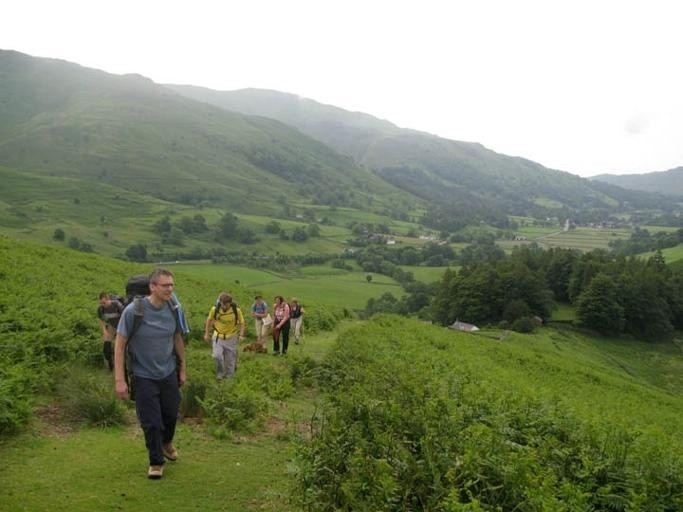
161 441 179 462
147 463 164 479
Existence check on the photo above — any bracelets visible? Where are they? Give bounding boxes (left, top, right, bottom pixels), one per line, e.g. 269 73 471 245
239 334 244 337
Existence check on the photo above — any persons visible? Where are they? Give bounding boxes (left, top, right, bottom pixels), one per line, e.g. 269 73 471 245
93 290 125 370
288 298 305 346
248 295 271 346
271 295 292 356
111 268 187 479
203 291 246 382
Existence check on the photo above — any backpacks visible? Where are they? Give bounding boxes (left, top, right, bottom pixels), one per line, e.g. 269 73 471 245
214 298 238 321
123 273 180 344
111 295 125 313
